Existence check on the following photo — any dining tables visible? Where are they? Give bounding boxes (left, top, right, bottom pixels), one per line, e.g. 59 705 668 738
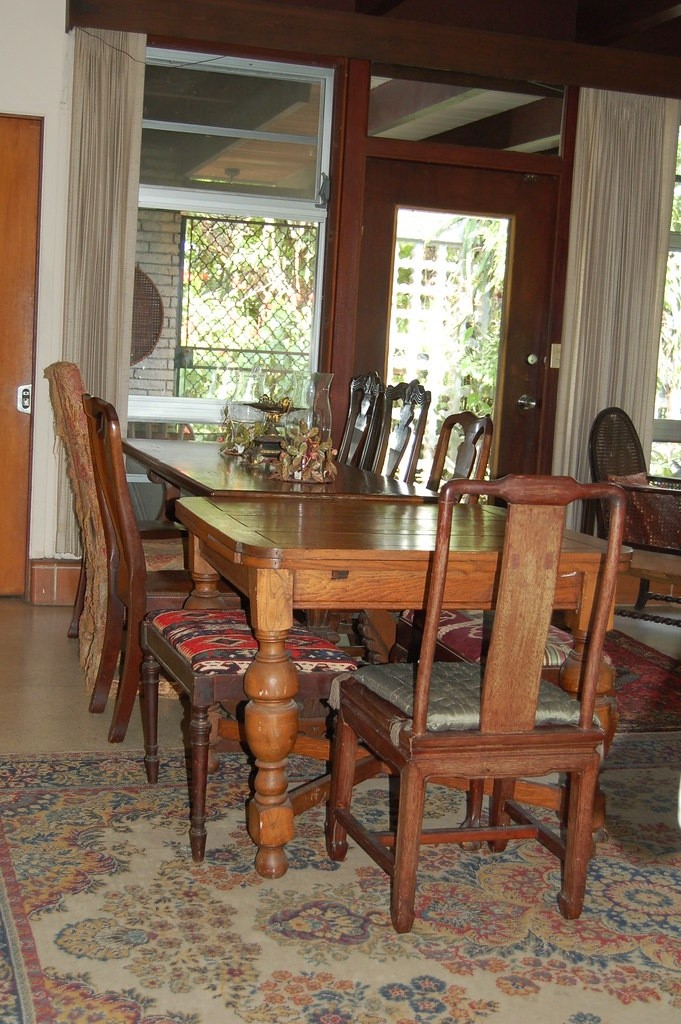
176 497 633 879
120 438 440 521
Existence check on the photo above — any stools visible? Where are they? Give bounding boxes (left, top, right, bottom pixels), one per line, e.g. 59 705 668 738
140 609 358 861
389 609 611 691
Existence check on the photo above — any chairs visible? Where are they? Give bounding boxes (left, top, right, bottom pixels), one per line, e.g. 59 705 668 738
325 474 627 933
82 393 247 742
425 412 493 505
336 370 385 471
66 520 188 636
590 407 681 627
42 361 188 699
371 379 432 484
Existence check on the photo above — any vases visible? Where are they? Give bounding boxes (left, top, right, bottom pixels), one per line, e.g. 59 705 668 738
284 371 335 483
224 371 268 455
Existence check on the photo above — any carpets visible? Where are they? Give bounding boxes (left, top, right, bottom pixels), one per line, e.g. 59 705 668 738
0 730 681 1024
605 628 681 731
